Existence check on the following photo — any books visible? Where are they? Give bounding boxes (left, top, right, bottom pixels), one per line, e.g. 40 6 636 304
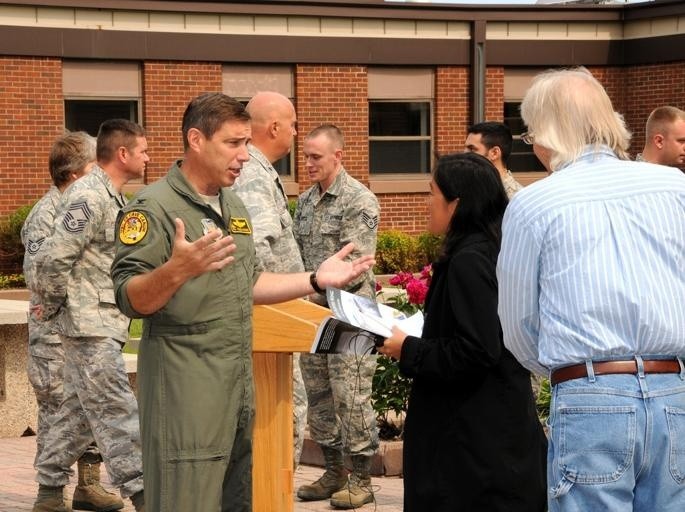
310 285 406 359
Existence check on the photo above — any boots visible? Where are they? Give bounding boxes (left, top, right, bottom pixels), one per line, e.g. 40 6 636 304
33 484 74 512
72 462 124 512
297 447 348 500
330 456 373 508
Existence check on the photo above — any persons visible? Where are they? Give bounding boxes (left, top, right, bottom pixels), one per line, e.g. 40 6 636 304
464 121 525 201
20 131 124 511
635 105 685 165
495 66 685 512
375 151 548 512
230 92 308 476
291 124 382 510
110 92 376 512
29 118 150 512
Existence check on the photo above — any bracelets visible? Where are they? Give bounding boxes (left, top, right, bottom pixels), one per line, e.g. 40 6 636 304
310 270 325 294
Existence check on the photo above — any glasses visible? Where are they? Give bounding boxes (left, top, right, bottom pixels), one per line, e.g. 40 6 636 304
521 132 533 145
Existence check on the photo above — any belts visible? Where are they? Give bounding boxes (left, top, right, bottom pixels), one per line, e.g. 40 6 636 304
551 360 685 387
